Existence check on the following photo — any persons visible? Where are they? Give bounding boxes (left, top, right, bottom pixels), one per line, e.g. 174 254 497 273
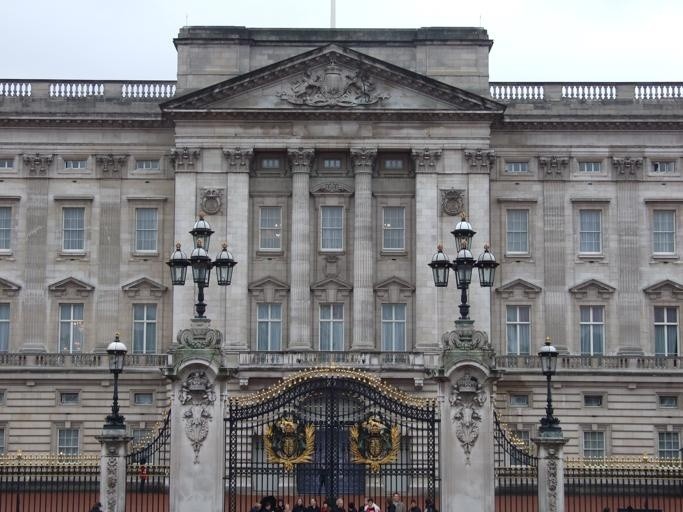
317 465 328 494
90 502 102 512
139 460 147 493
250 493 436 512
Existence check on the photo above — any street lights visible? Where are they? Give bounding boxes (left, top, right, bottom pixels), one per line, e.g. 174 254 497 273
428 211 499 324
103 332 126 429
538 336 561 432
166 211 238 322
